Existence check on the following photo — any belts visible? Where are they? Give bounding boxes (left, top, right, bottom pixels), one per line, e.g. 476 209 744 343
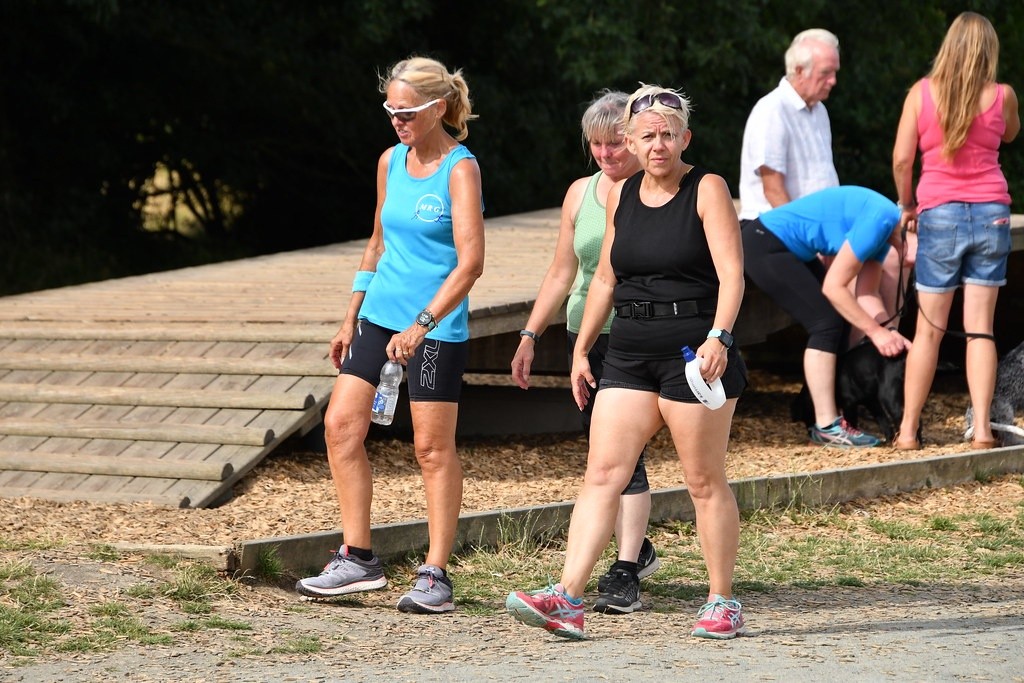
616 301 713 319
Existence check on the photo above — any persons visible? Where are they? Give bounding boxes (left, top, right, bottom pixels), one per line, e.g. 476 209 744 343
892 11 1019 450
740 185 912 449
740 28 917 351
296 57 486 614
505 83 748 639
511 92 659 614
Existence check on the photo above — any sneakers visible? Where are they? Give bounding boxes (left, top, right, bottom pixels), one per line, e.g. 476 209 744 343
811 416 881 449
295 544 388 597
691 593 745 639
592 561 642 613
396 564 455 613
506 583 584 640
597 536 659 594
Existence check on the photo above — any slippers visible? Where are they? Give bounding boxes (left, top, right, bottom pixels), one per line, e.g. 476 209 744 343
892 431 921 450
970 429 1002 449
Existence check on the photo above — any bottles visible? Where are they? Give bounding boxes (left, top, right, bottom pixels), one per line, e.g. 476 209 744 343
371 354 404 426
682 345 726 411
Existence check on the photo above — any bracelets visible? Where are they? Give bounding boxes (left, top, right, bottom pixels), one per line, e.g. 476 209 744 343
889 326 896 332
520 330 539 345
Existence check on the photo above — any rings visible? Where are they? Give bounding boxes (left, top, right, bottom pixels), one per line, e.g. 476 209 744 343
403 352 409 355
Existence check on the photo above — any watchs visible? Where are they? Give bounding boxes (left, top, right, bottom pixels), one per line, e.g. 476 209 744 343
416 311 436 331
706 329 733 350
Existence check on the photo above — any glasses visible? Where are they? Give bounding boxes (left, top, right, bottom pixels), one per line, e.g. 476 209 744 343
629 93 682 120
383 92 451 121
